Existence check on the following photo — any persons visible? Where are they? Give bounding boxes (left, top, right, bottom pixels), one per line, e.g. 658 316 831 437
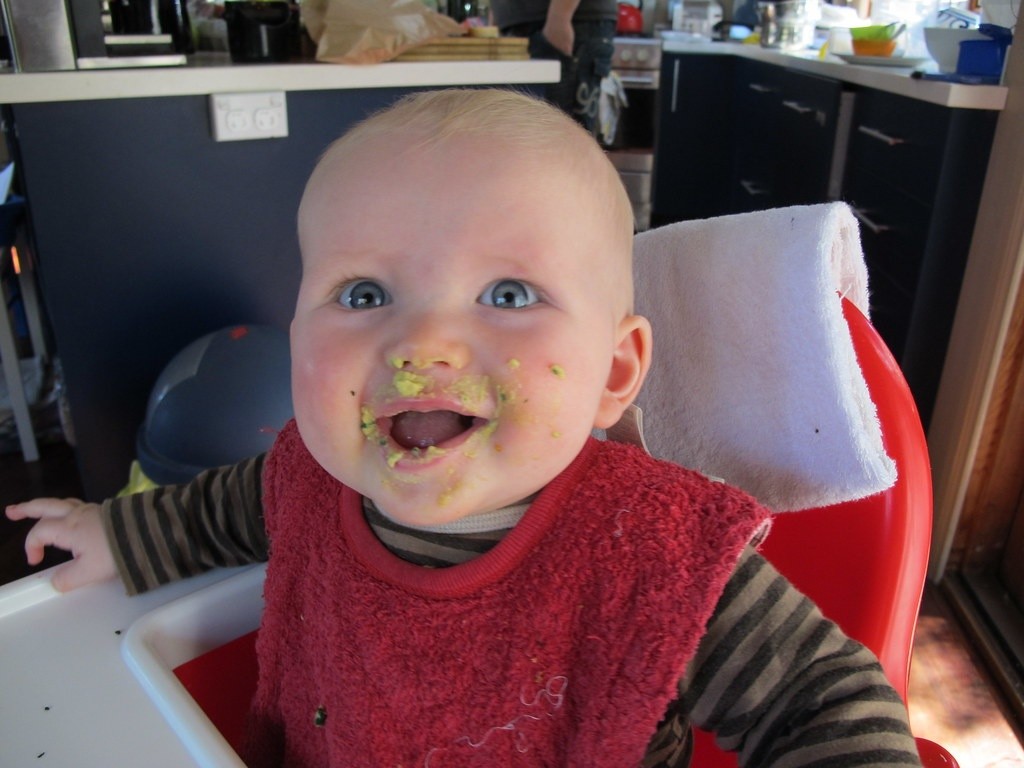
488 0 618 134
4 88 923 767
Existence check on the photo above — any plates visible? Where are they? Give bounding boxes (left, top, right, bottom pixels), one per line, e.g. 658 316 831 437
830 52 933 69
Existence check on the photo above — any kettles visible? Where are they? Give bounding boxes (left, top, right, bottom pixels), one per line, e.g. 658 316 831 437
616 0 645 35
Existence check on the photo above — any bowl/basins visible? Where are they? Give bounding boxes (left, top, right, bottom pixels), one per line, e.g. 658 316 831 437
850 39 899 55
924 26 994 74
753 0 818 50
849 26 896 40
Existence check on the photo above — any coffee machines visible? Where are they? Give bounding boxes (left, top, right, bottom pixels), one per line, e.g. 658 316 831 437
1 0 189 73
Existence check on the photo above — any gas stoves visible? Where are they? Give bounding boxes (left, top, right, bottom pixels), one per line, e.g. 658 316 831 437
604 29 664 68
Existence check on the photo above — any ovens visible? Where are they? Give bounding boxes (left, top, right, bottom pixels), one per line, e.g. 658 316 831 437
565 67 660 172
